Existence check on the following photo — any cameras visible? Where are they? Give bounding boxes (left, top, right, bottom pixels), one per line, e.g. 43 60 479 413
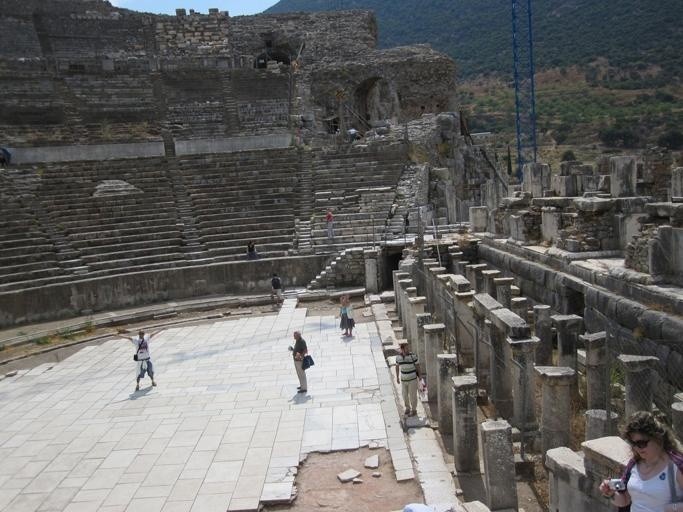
607 479 625 491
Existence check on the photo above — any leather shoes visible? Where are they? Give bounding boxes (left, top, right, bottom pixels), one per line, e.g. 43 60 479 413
405 409 417 417
297 387 307 393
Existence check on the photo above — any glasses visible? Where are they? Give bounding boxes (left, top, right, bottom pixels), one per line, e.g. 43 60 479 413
629 439 651 449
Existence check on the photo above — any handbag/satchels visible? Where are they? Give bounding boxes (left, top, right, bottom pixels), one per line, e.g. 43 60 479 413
302 355 315 370
134 354 138 361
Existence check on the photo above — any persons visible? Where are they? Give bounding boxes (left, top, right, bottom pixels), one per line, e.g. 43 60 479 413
396 343 423 417
289 331 309 393
325 207 334 239
118 328 166 391
348 125 359 143
339 296 355 337
248 240 256 256
599 411 683 512
271 273 282 304
